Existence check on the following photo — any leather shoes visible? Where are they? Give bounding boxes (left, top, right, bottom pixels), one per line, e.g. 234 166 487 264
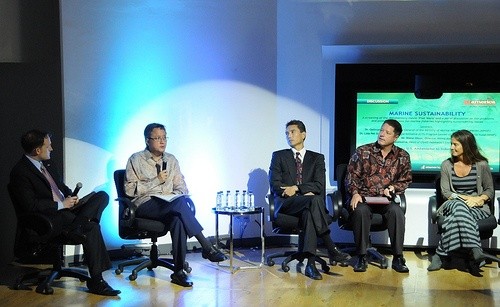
171 272 193 287
392 256 410 273
328 245 352 262
305 263 323 280
201 246 227 262
354 256 368 271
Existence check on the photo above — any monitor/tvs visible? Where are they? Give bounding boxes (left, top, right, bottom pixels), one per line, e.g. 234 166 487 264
334 63 500 191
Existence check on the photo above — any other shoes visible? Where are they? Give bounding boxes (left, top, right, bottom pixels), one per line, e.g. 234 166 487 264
89 280 122 296
71 215 94 232
472 248 487 264
427 255 443 270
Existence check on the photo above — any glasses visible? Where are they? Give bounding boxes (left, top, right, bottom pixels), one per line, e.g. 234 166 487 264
150 137 169 142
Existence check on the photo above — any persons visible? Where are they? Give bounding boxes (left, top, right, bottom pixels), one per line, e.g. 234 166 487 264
124 123 228 286
426 130 495 271
268 120 352 281
10 129 121 296
345 119 411 272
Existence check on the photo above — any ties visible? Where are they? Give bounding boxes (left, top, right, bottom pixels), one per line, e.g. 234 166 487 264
296 152 303 185
41 165 64 202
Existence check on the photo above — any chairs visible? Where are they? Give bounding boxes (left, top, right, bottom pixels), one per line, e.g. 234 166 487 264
430 177 500 269
267 163 406 274
113 170 192 280
6 186 91 295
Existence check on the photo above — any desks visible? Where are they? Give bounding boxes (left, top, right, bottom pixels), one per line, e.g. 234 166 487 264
212 207 264 270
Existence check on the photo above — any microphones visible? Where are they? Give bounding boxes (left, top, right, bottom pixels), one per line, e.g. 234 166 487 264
70 183 82 197
162 156 168 171
388 188 401 203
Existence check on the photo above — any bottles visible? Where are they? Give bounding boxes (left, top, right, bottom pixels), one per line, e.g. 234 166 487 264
219 191 225 210
234 190 241 211
216 192 222 210
241 190 247 211
247 190 255 211
225 191 232 209
